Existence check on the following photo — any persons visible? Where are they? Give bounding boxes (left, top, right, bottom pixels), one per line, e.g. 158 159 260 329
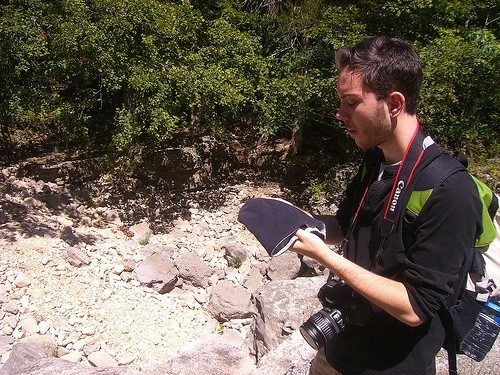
288 35 483 375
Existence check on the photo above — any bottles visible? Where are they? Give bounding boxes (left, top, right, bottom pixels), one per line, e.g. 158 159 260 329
460 287 500 362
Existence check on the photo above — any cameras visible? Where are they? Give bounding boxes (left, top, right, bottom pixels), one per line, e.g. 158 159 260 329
299 278 376 350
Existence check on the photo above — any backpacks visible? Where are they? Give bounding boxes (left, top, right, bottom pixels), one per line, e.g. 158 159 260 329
360 151 500 355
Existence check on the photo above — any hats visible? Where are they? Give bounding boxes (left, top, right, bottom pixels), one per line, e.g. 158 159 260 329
238 197 326 257
